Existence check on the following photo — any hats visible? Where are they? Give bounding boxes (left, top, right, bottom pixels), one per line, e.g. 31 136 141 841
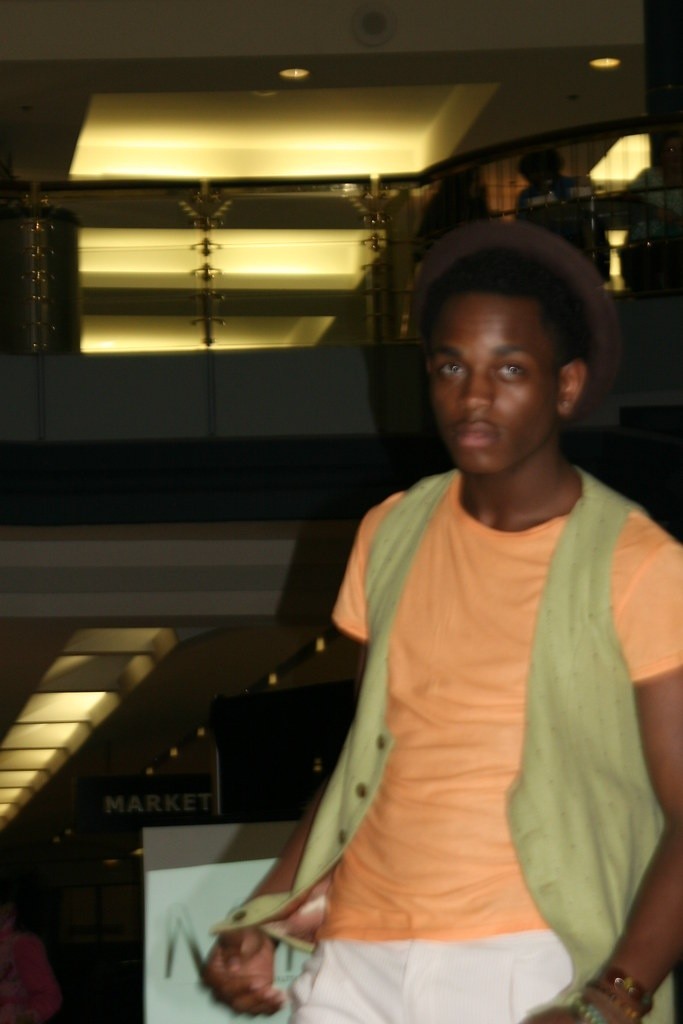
407 219 620 422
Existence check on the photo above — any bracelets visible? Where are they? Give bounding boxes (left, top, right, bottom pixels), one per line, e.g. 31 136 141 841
570 999 606 1024
586 980 642 1020
598 966 654 1012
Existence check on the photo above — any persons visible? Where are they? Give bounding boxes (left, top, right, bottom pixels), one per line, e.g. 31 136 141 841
200 222 681 1024
0 909 62 1024
